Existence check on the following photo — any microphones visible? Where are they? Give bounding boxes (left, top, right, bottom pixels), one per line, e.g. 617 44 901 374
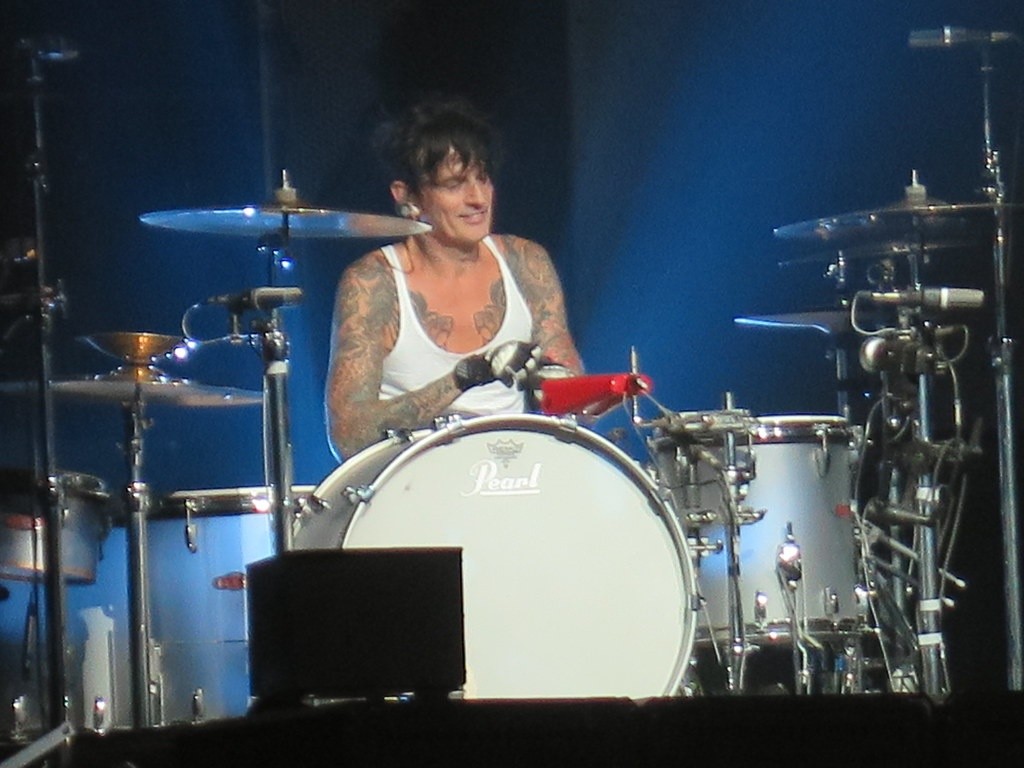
864 286 983 310
204 284 301 313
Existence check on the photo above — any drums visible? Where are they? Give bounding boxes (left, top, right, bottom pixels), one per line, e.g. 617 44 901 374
0 465 109 587
0 485 317 736
650 414 876 647
289 410 703 697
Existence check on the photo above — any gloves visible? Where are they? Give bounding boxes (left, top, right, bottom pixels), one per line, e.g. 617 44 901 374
517 359 577 413
454 339 552 391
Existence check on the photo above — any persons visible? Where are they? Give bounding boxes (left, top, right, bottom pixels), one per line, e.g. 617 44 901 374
321 103 590 467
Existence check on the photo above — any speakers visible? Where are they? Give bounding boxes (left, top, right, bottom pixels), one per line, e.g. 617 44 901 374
241 544 465 712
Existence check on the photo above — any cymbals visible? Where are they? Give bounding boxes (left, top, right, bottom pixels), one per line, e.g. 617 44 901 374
733 295 902 333
140 201 434 239
47 332 264 406
771 197 1024 269
